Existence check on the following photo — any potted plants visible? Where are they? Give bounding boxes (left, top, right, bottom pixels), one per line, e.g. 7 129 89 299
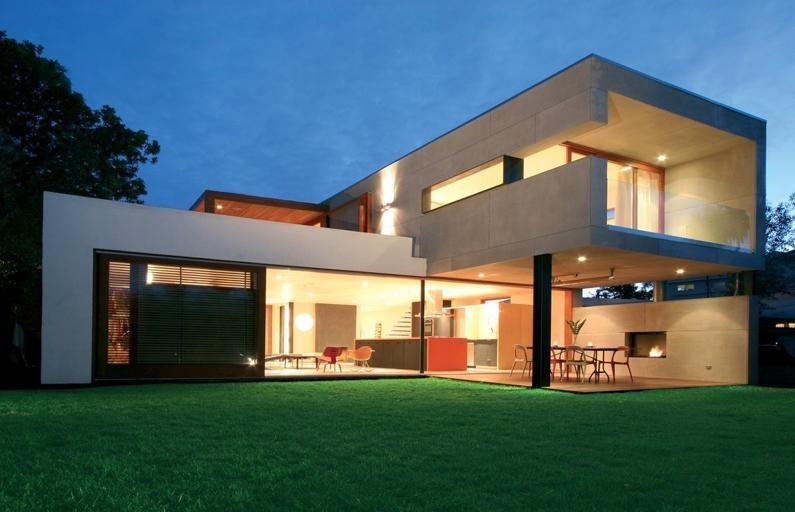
564 318 588 344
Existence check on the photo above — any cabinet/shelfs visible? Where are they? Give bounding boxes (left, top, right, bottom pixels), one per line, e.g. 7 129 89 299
351 301 533 373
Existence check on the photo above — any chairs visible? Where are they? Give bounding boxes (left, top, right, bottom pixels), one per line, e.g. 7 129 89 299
507 343 634 386
276 342 377 374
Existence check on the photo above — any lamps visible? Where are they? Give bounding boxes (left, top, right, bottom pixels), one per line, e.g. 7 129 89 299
551 264 616 290
